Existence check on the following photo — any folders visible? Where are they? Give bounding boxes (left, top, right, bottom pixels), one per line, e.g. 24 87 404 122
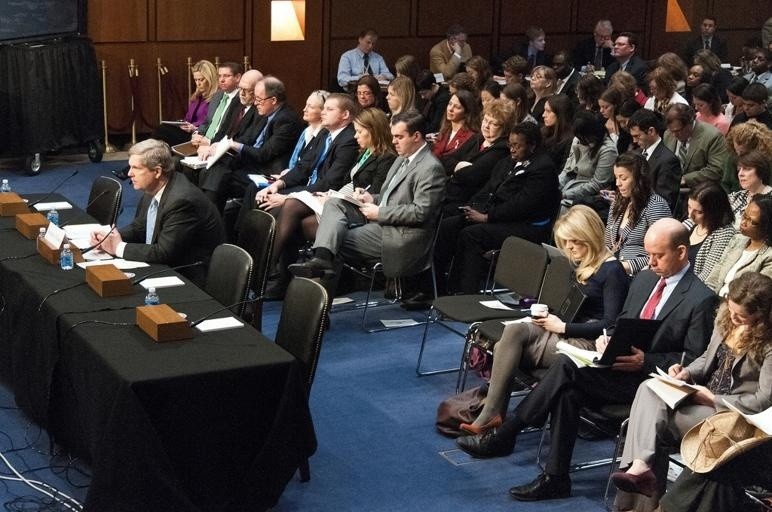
600 318 663 363
558 282 589 323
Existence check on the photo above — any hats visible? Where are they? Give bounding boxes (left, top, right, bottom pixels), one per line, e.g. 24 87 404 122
680 409 768 474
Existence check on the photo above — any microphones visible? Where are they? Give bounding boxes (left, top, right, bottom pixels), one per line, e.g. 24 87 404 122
132 260 205 285
28 169 79 207
58 187 112 227
188 296 263 329
81 224 126 255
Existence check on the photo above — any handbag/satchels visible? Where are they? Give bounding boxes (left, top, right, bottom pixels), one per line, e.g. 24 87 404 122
435 386 487 437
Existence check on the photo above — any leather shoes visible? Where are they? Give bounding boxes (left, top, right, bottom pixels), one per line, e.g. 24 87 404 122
263 283 289 301
456 430 515 459
287 259 335 279
509 474 571 501
401 290 448 309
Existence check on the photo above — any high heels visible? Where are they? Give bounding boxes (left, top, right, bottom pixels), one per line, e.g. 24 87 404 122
112 167 135 185
460 415 502 435
612 469 657 498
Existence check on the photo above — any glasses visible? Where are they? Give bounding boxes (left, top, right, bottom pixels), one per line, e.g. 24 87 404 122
507 142 531 151
483 118 501 126
236 86 254 93
356 91 373 95
253 96 275 104
314 87 326 102
740 209 763 225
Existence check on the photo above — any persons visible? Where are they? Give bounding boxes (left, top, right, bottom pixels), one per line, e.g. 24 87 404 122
606 70 646 107
686 180 738 283
527 63 561 120
691 83 729 135
685 62 713 103
723 76 750 121
644 67 690 114
429 24 473 78
430 89 482 158
725 152 771 229
720 118 772 192
453 217 719 504
90 139 227 288
660 101 731 211
604 31 649 95
761 16 772 49
574 74 604 125
539 92 580 174
230 92 361 230
401 120 561 311
724 82 772 140
288 88 334 171
599 108 683 220
262 107 402 300
171 60 245 178
656 51 688 98
551 49 583 101
199 76 303 216
704 192 771 298
607 270 772 511
518 25 555 72
448 71 479 103
354 75 387 112
503 53 534 104
183 70 264 192
413 70 453 133
480 80 503 108
395 54 418 79
458 203 629 438
598 86 622 144
613 98 644 155
110 60 220 185
556 110 618 216
337 26 394 92
692 49 732 103
686 16 728 62
465 54 493 86
287 110 447 332
602 152 675 280
653 444 772 512
498 83 538 124
573 18 618 70
437 97 519 222
384 77 420 124
742 48 772 94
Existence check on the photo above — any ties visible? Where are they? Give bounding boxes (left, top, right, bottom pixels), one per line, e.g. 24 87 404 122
363 55 373 76
595 46 602 71
379 159 409 210
145 197 159 244
205 93 229 139
750 75 758 85
705 40 710 52
308 134 333 186
641 278 667 320
527 55 533 73
228 106 245 138
642 151 648 160
679 141 687 177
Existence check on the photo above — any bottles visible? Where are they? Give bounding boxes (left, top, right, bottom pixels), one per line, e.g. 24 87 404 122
37 228 46 249
0 179 11 192
47 207 59 226
61 244 73 270
145 288 159 305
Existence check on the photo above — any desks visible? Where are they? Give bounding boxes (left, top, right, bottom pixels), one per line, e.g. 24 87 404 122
348 81 530 111
0 192 318 512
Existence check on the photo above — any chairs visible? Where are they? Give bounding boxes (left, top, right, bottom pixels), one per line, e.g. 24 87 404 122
669 457 686 469
446 189 563 296
415 235 547 395
460 256 575 397
512 370 629 501
239 209 276 333
204 243 254 318
344 208 447 333
87 175 123 225
275 277 328 483
603 417 631 511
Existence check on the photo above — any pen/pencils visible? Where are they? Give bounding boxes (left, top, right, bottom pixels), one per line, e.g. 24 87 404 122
603 328 607 345
680 352 685 370
587 62 591 68
361 185 371 194
